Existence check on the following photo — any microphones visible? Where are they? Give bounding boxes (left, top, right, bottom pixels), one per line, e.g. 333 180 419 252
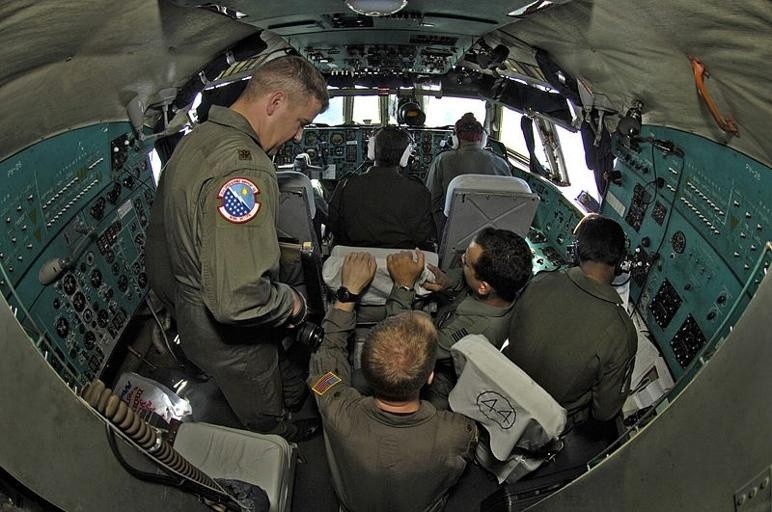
609 273 634 292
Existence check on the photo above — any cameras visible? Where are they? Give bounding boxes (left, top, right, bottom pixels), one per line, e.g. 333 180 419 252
280 325 325 351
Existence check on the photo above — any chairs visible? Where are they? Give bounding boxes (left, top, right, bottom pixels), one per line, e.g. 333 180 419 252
438 174 540 273
450 333 566 483
276 173 322 268
322 244 438 323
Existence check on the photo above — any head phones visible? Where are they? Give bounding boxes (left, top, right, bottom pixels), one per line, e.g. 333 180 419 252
568 212 637 274
365 121 416 167
448 128 490 149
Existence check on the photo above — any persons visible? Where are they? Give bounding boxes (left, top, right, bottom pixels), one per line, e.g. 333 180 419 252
144 54 329 442
328 125 437 249
426 113 512 248
